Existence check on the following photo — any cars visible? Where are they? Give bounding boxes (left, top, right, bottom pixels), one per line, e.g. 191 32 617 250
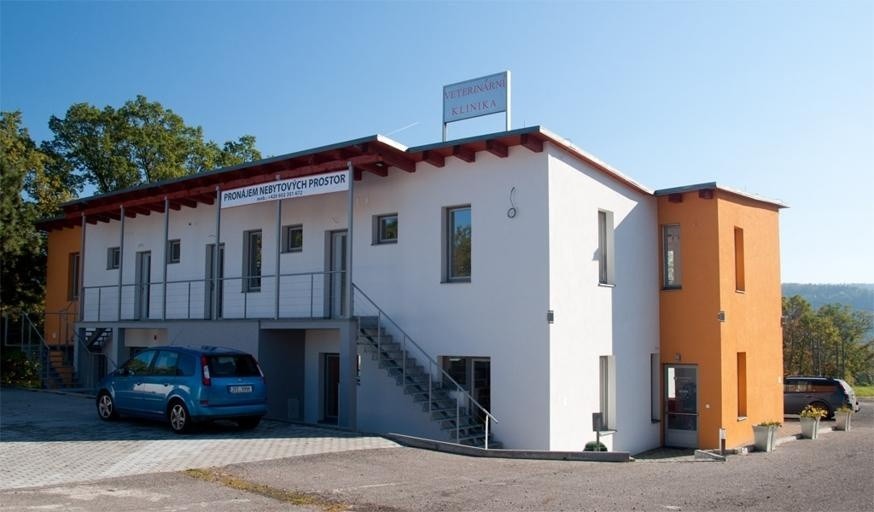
94 344 270 434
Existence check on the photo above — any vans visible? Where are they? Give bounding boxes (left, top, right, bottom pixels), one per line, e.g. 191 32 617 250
784 376 861 422
